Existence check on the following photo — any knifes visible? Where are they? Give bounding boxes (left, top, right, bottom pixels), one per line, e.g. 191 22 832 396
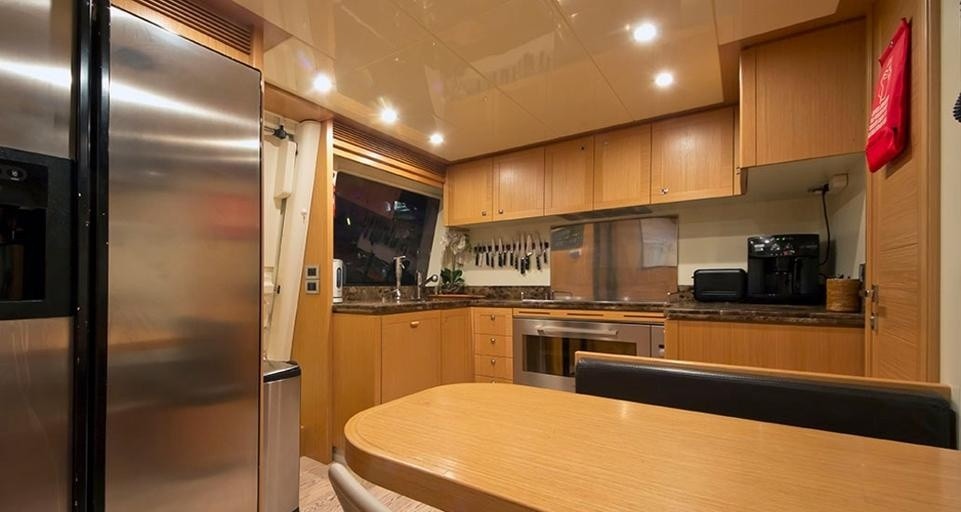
474 231 547 274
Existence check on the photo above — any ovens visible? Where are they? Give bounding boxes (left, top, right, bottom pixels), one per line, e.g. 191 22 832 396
512 316 665 393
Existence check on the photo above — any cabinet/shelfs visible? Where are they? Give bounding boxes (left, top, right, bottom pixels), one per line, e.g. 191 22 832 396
652 104 735 203
473 309 514 383
547 123 652 216
446 144 544 226
331 307 473 447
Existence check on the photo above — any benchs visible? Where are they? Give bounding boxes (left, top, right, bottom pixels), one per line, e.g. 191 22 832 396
575 352 956 451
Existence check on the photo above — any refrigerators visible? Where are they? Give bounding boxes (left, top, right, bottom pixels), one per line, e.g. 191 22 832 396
0 0 263 512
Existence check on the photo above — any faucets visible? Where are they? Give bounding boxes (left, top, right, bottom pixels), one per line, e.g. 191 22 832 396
392 256 406 288
416 272 438 301
381 289 401 301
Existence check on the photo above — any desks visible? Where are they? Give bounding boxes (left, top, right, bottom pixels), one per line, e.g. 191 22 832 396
344 381 961 511
